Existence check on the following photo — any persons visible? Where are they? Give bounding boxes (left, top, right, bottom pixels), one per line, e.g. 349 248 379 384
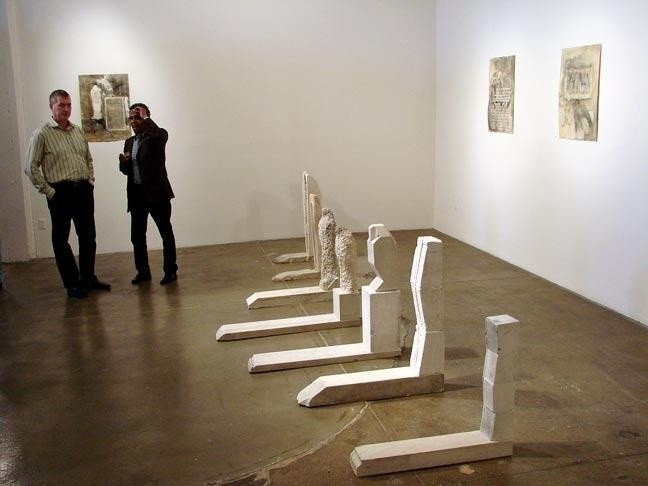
24 89 112 299
118 103 178 285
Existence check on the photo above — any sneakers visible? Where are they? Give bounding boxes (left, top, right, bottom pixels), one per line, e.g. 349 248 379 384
160 272 178 285
67 276 111 297
130 271 151 284
